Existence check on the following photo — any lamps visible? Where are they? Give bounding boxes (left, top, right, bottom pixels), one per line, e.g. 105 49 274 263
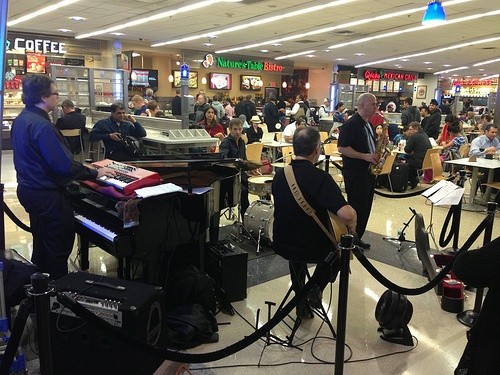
275 1 500 60
75 0 218 40
7 0 80 27
421 0 446 27
354 33 500 68
215 0 473 54
151 0 358 47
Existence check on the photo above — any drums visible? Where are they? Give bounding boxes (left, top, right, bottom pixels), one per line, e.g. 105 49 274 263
247 175 274 195
243 200 274 246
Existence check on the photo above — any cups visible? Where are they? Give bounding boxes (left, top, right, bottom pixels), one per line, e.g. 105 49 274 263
479 145 485 152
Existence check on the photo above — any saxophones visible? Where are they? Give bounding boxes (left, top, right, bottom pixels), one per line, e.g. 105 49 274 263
368 112 394 175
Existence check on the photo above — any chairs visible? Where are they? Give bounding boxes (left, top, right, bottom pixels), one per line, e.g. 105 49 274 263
60 129 85 164
226 116 500 217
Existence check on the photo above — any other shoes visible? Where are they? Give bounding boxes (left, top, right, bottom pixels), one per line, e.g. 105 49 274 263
357 240 371 249
305 282 322 309
410 178 419 189
296 298 314 319
447 176 456 181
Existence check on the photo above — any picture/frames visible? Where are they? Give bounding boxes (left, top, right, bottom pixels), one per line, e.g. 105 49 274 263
416 85 427 99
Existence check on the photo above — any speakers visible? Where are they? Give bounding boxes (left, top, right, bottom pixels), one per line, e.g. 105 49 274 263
207 242 247 303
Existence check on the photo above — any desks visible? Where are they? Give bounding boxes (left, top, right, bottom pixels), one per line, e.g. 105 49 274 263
264 142 293 172
465 131 480 143
317 155 343 170
444 157 500 211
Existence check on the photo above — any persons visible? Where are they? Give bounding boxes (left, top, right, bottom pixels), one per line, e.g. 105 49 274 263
452 236 500 375
171 89 181 115
337 93 378 249
54 100 86 155
11 74 116 280
88 102 146 161
271 126 357 318
127 88 160 118
195 91 500 221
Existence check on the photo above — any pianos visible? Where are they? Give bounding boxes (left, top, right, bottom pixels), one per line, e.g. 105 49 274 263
74 157 241 279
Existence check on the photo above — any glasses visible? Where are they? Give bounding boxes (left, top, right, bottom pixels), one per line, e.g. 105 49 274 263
43 91 59 97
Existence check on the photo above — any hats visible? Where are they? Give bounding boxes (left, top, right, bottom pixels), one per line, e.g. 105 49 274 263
430 99 439 106
250 115 262 123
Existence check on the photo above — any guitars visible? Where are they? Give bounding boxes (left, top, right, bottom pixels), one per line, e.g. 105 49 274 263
326 208 353 261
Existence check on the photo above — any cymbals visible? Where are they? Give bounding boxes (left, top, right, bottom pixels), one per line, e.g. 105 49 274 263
270 163 289 168
241 160 263 171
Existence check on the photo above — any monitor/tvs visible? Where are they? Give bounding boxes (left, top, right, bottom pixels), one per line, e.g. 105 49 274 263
209 72 231 90
240 75 262 91
131 69 158 89
172 70 198 89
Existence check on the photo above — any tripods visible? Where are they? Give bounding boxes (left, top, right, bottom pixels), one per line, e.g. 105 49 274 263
221 168 262 249
383 200 438 254
